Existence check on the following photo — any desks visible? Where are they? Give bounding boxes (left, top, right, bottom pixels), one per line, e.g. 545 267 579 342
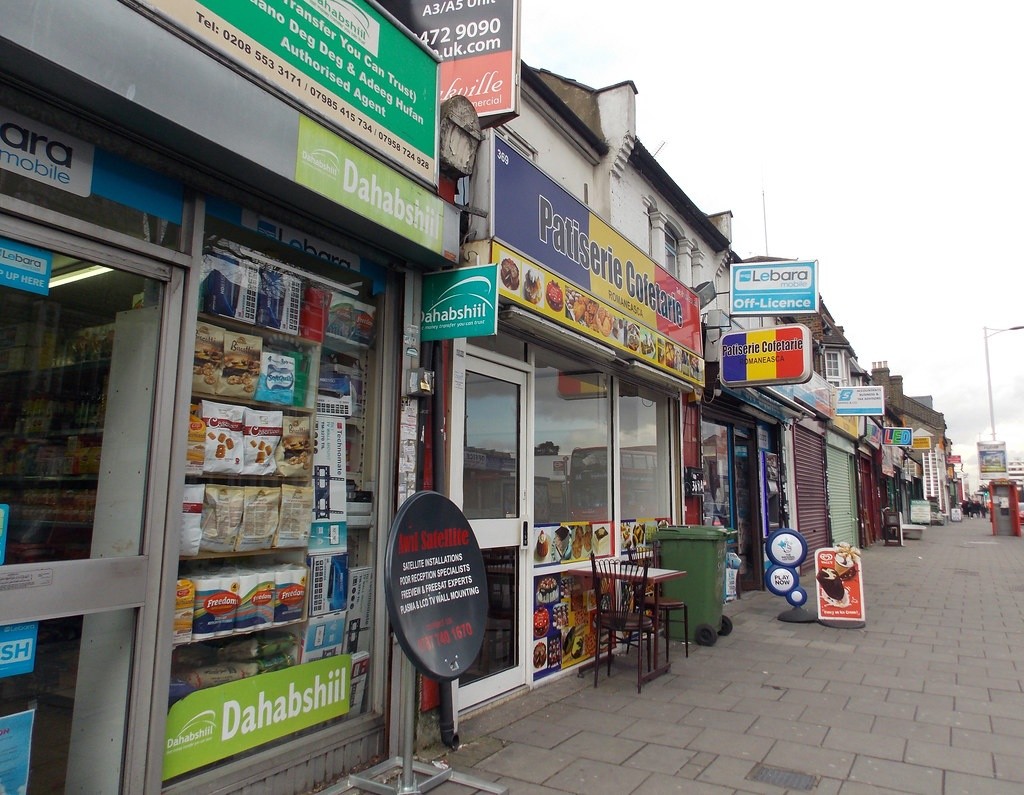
566 562 685 686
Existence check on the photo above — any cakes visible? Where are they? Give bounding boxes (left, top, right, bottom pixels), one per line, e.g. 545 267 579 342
534 579 611 667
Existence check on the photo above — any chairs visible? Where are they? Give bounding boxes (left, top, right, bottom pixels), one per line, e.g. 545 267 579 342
590 550 654 692
626 543 689 664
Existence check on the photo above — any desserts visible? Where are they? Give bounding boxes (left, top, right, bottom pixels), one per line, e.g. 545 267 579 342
537 527 608 560
547 280 564 310
525 270 542 303
501 257 519 289
566 298 701 371
622 522 658 549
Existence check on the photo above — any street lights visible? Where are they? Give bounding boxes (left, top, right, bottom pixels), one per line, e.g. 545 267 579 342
983 324 1024 440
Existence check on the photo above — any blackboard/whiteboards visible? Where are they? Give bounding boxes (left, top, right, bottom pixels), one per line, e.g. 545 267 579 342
951 508 962 522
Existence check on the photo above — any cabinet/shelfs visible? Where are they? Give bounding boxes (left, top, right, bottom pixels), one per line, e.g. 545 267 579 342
0 354 114 557
169 313 324 652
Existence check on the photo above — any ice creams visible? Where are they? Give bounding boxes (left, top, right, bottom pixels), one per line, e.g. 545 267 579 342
817 544 860 600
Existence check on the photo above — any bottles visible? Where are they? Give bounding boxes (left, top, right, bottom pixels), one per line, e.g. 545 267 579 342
20 393 107 432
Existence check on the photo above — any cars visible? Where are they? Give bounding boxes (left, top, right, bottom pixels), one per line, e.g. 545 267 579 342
1018 502 1024 525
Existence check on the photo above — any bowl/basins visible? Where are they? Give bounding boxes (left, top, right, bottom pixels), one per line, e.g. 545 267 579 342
892 524 926 539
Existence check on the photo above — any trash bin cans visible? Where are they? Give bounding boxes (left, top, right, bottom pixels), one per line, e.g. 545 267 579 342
650 524 733 647
930 502 939 525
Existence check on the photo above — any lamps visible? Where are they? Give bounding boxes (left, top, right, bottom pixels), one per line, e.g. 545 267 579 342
693 281 717 310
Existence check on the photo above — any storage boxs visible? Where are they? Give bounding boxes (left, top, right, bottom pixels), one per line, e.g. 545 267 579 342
301 415 370 720
199 253 260 324
313 379 355 417
254 262 303 336
